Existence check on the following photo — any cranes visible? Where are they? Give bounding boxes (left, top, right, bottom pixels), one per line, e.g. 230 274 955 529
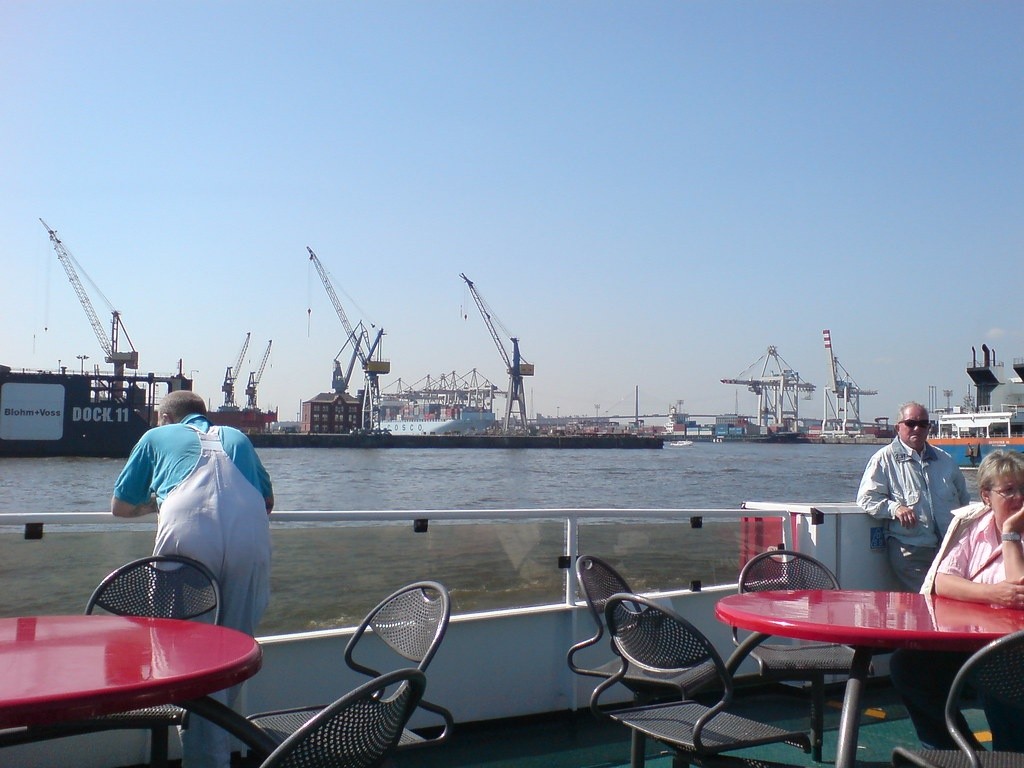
306 245 390 436
245 339 273 413
218 331 251 412
718 346 817 432
822 328 878 435
459 273 534 435
39 216 138 401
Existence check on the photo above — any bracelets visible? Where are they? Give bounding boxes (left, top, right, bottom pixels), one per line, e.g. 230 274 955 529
1001 532 1021 543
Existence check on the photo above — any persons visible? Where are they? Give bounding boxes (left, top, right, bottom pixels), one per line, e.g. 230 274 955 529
888 449 1024 750
855 401 971 593
111 390 274 768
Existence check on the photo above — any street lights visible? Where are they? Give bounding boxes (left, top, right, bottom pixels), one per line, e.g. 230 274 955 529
75 354 89 375
593 403 600 426
556 407 559 427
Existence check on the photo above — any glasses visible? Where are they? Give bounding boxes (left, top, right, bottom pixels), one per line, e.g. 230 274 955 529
987 485 1024 500
898 420 930 429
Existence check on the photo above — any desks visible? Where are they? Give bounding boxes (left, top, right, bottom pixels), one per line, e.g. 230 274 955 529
714 589 1024 768
0 614 281 768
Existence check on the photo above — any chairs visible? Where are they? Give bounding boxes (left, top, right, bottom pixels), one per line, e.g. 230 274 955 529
241 580 454 759
892 629 1024 768
567 555 717 701
732 550 874 762
258 668 427 768
26 555 223 730
591 592 812 768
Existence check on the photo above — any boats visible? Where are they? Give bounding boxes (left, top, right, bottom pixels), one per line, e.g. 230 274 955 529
662 441 694 449
927 437 1024 468
